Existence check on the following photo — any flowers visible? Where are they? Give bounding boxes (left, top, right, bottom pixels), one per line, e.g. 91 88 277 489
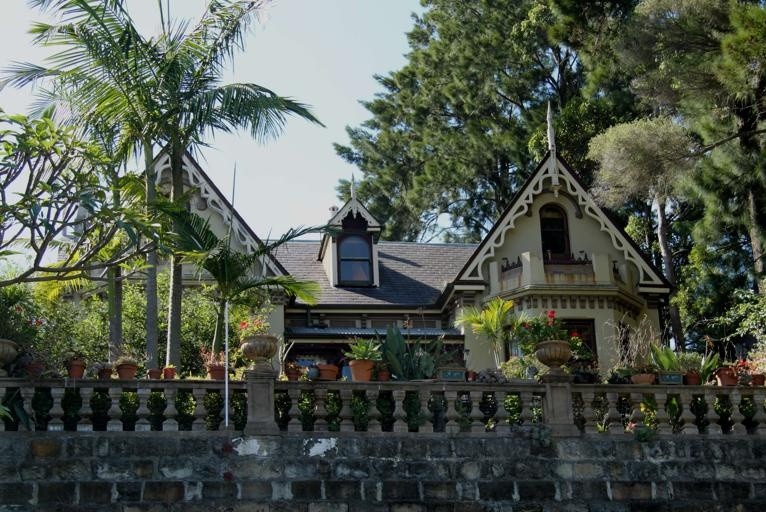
0 298 44 349
233 304 274 338
521 306 565 340
202 349 225 369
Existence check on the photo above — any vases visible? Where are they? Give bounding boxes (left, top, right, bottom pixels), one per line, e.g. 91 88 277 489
239 336 280 370
0 339 20 380
316 364 340 382
206 366 225 381
536 340 573 377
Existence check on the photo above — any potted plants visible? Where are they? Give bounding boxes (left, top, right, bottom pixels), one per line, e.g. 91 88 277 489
617 341 766 388
63 343 175 379
287 362 300 379
342 332 392 382
